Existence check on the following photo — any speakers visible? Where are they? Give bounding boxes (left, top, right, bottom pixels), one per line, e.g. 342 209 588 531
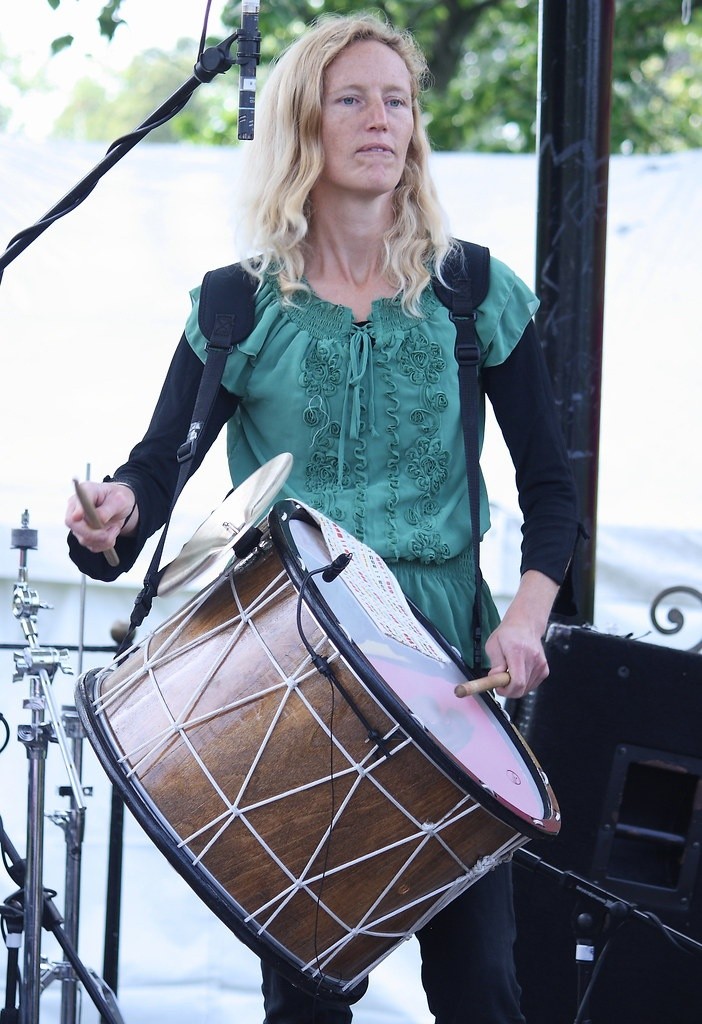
500 620 702 1023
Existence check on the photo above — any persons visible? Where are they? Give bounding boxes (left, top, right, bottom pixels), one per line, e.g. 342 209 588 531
65 23 587 1023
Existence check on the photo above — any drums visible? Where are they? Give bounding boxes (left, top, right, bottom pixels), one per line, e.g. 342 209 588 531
72 497 563 1003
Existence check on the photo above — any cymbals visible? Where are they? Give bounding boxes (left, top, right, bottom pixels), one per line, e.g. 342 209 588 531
152 446 294 597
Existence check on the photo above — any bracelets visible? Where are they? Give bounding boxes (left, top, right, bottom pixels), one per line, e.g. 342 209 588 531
116 484 137 531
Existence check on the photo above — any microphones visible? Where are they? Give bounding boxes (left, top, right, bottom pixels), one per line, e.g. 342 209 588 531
237 1 260 142
321 552 353 584
